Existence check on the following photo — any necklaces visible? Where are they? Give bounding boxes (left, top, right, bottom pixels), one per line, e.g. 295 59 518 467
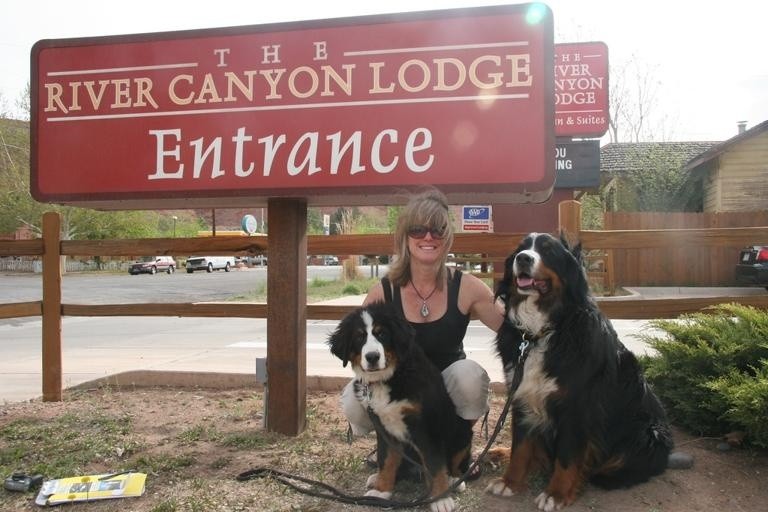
409 274 438 317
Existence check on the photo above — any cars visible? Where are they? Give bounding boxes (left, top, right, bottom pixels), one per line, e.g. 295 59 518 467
735 245 768 291
237 255 268 265
307 255 324 266
128 256 176 275
186 256 235 273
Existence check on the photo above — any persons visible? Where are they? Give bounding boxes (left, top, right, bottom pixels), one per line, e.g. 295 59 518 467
360 191 508 480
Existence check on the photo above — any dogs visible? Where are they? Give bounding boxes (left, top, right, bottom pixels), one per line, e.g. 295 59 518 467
485 230 677 512
321 297 514 511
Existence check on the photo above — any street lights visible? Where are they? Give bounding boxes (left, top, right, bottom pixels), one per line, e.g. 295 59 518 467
172 216 178 237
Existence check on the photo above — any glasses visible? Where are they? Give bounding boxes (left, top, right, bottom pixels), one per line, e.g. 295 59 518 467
407 224 449 239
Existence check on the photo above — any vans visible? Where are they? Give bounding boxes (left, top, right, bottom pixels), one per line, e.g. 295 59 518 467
326 256 339 265
